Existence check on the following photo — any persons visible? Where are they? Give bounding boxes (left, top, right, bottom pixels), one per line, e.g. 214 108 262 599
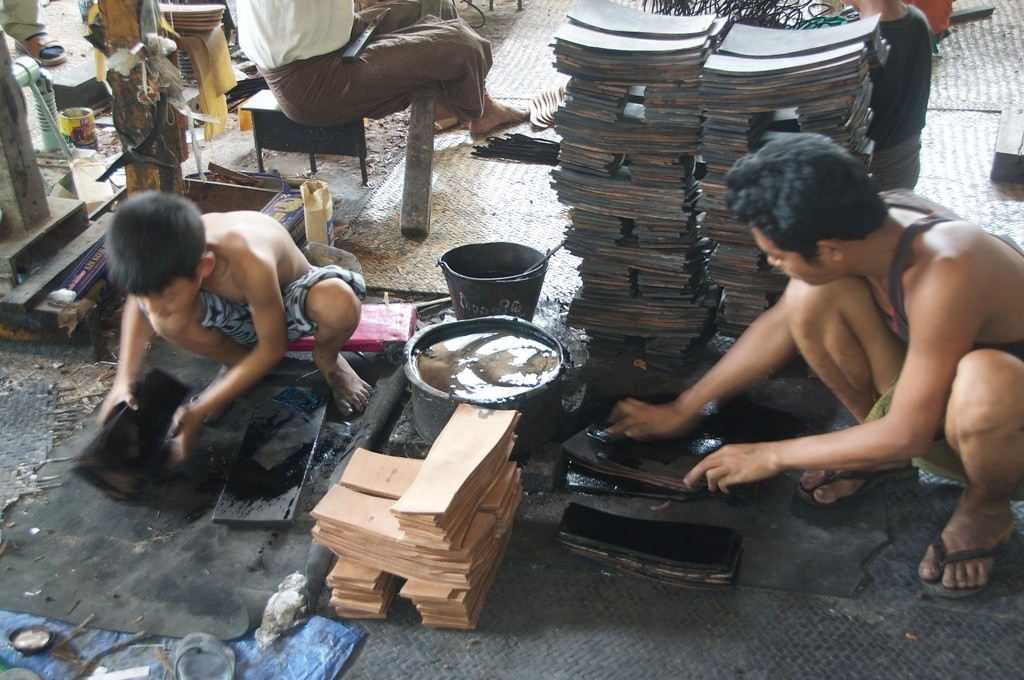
97 189 374 471
226 0 531 141
842 0 933 192
604 133 1020 599
0 0 66 66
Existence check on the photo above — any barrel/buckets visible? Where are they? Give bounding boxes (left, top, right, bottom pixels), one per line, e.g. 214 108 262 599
438 242 549 323
403 314 566 445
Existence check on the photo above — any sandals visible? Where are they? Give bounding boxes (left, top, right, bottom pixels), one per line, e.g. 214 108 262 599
15 33 66 66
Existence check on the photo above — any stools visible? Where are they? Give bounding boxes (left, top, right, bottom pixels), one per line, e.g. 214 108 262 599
287 301 417 364
240 88 369 185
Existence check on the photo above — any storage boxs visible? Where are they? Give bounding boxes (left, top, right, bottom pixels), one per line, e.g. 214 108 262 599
52 58 111 111
264 191 307 247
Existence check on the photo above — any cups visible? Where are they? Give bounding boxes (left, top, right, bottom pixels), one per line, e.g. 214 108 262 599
59 106 98 152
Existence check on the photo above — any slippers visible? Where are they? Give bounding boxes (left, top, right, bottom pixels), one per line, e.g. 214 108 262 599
796 463 918 511
173 632 235 680
919 502 1014 598
